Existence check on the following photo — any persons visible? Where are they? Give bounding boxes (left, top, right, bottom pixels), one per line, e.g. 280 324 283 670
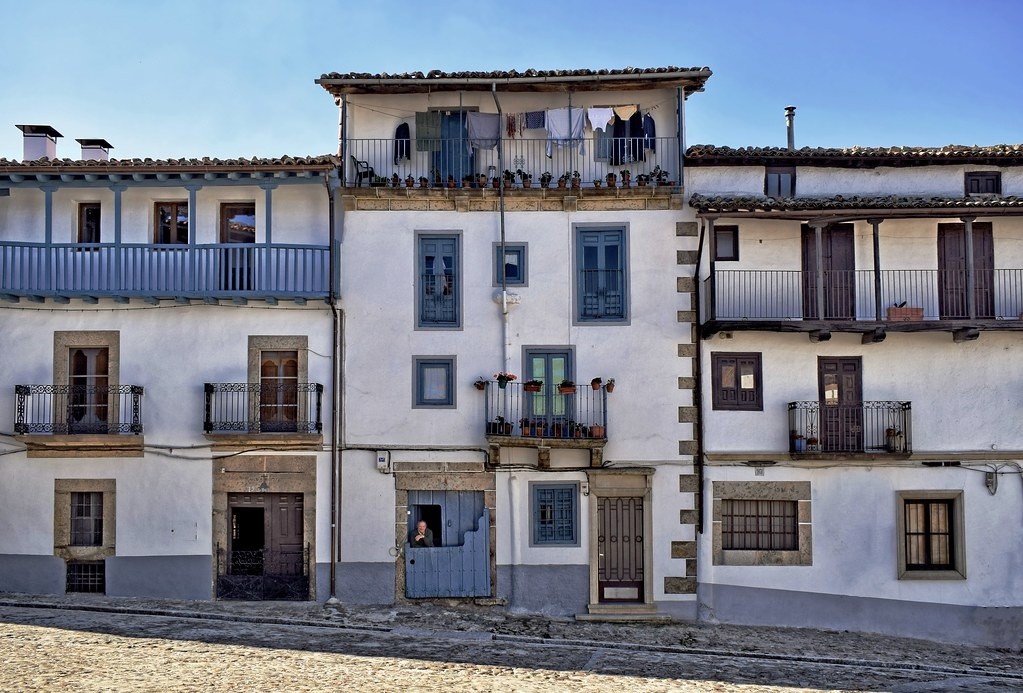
410 520 435 548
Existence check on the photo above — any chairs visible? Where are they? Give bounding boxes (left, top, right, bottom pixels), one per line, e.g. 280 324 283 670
351 155 376 188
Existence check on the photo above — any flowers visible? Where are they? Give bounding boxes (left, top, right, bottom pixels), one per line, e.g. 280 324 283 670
568 420 583 438
473 375 490 385
495 416 504 424
525 377 544 386
494 371 517 381
519 418 548 428
551 422 561 431
557 380 574 388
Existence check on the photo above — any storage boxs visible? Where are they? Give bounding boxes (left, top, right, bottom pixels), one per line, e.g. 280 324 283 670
887 307 924 321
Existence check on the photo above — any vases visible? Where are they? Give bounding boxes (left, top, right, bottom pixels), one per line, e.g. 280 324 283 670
668 181 674 185
559 387 576 394
536 427 545 436
575 431 581 437
553 431 559 436
488 422 513 434
522 427 531 436
478 383 484 390
500 381 508 389
524 385 541 391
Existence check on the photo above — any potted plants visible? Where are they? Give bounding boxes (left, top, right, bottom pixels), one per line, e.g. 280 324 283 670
791 430 822 452
537 171 555 188
620 170 631 180
491 176 501 187
369 175 391 188
590 425 605 437
635 166 669 186
392 173 402 188
448 174 458 188
430 164 444 187
406 174 415 187
886 427 906 451
607 378 615 393
557 170 582 188
503 169 533 189
607 173 617 187
593 179 602 187
462 174 489 188
591 377 602 390
418 175 429 187
